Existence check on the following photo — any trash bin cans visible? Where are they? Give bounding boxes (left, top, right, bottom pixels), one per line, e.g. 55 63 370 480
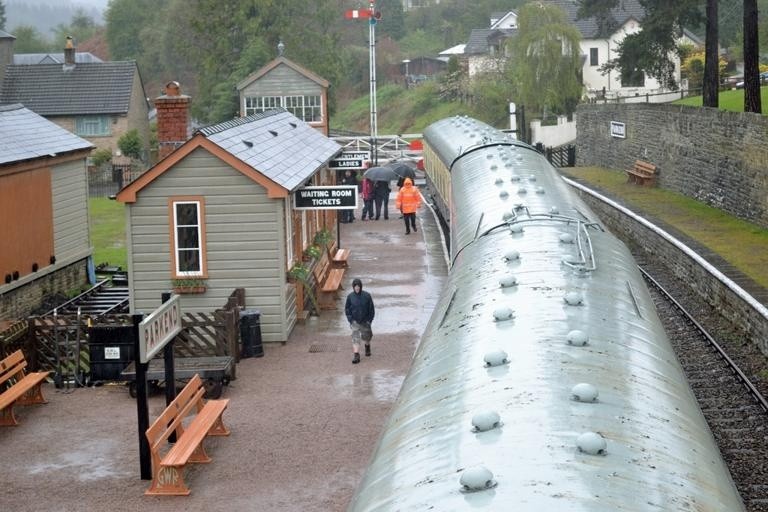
239 309 264 358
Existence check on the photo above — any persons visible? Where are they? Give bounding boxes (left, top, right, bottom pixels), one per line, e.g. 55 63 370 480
342 170 414 224
396 178 422 235
345 279 374 364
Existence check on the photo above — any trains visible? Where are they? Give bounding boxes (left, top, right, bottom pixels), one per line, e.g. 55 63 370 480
349 116 747 511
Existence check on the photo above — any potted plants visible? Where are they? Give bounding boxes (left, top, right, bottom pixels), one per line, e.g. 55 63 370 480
288 226 332 284
170 272 208 295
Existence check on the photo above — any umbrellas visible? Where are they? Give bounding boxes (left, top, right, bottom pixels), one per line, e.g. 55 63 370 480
388 162 416 179
364 166 396 182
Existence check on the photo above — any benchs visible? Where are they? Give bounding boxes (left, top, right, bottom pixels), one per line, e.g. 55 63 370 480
143 372 232 497
0 348 52 428
313 237 351 312
624 159 658 188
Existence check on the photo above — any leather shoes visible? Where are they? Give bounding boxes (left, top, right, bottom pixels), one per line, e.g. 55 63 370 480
364 343 371 356
352 352 360 363
